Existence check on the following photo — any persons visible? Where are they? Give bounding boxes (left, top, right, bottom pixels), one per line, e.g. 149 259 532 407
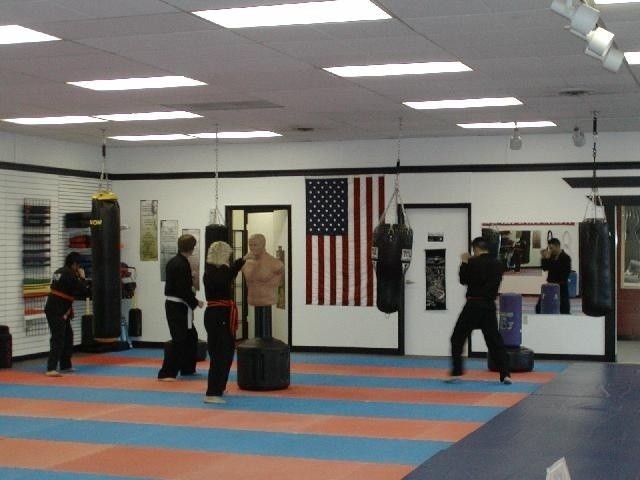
42 250 86 377
156 233 204 380
240 233 285 307
202 240 255 404
120 261 137 348
443 236 513 386
534 237 571 313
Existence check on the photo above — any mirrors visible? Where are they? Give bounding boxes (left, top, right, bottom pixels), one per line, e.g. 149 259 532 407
479 222 589 319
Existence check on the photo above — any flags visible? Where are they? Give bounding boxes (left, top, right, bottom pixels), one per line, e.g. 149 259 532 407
305 175 386 307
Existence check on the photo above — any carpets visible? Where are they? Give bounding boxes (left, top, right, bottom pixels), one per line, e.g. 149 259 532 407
2 340 575 478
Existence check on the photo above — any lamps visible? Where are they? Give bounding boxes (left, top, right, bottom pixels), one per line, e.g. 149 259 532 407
571 125 586 149
549 0 629 77
507 116 523 152
